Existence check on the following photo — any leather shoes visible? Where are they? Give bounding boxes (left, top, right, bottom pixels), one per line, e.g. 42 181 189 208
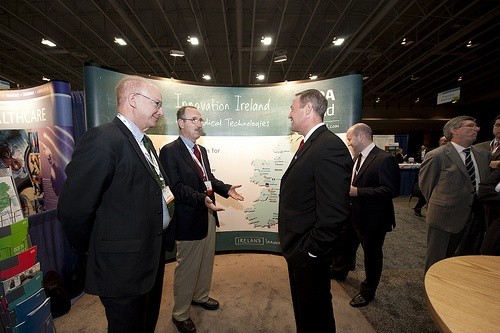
171 315 196 333
331 266 348 281
191 298 219 311
350 293 374 307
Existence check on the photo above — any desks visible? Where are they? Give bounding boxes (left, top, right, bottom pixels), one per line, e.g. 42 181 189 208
422 255 500 333
398 163 423 203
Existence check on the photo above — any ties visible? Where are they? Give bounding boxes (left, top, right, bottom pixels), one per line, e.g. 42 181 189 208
352 153 363 185
296 139 304 155
193 144 207 182
463 148 476 196
143 135 175 218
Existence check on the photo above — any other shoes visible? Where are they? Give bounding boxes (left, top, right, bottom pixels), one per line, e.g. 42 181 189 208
414 208 423 217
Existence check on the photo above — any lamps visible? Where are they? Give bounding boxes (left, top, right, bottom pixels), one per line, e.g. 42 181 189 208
35 0 345 82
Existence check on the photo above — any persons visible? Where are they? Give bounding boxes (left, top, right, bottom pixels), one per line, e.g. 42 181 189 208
159 106 244 333
57 76 176 333
328 124 401 308
279 89 354 333
392 115 500 275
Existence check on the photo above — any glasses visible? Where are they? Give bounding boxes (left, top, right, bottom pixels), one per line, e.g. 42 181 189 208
459 124 481 129
136 92 163 109
182 117 205 125
